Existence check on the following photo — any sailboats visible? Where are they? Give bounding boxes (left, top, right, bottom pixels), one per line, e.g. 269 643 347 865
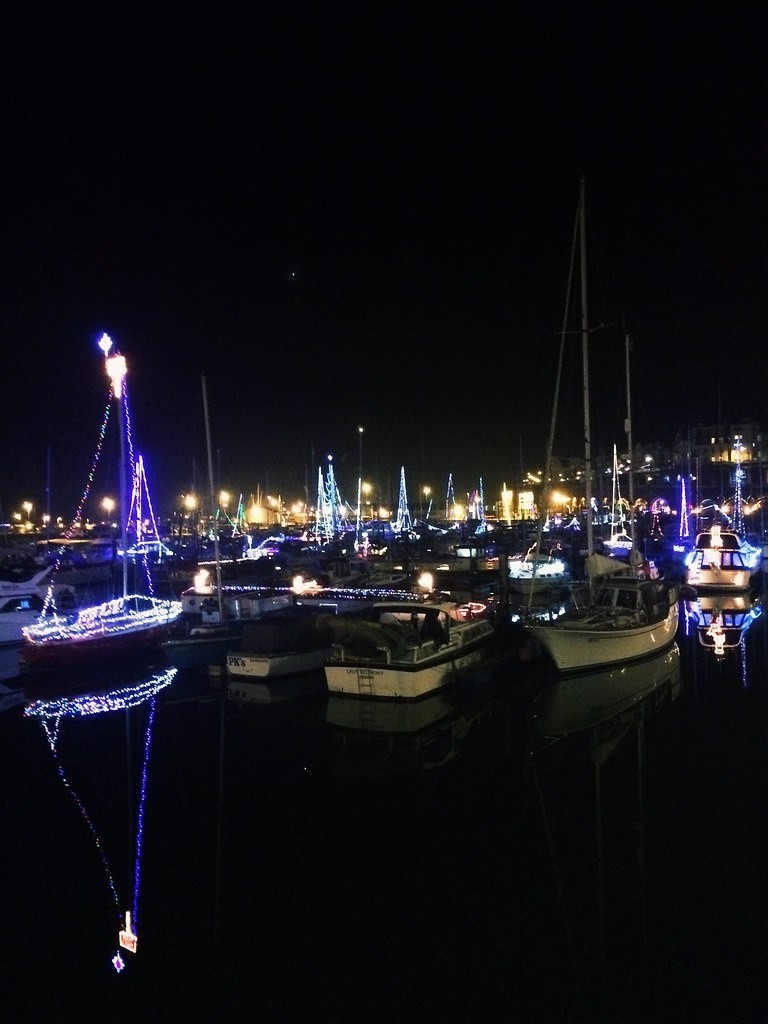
522 640 681 964
23 651 179 979
0 172 767 684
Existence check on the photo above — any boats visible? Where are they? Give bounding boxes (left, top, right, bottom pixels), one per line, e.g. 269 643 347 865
322 665 494 837
